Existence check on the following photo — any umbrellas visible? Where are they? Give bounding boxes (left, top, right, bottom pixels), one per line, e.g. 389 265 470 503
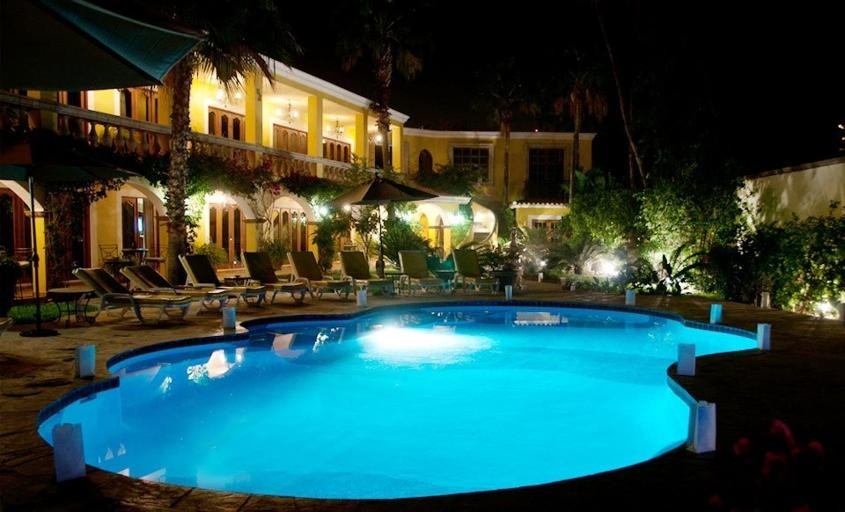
1 0 210 88
1 125 139 333
322 171 440 260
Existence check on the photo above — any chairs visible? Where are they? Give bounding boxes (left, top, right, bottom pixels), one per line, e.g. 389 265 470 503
71 242 503 328
